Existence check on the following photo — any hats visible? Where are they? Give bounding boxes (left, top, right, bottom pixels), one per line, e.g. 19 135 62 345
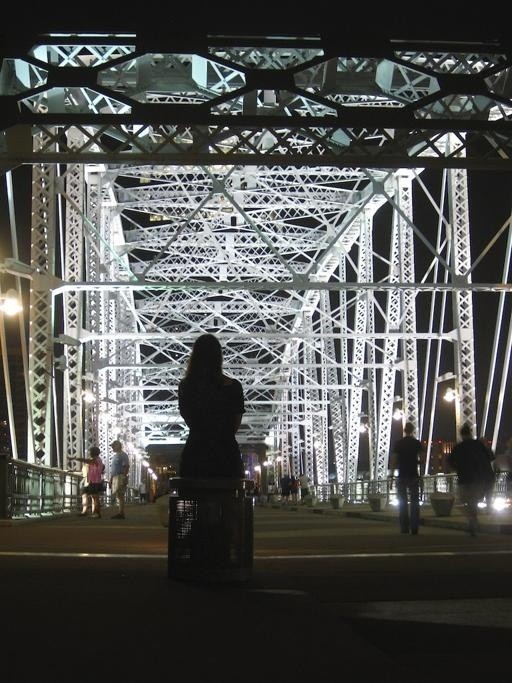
109 441 121 446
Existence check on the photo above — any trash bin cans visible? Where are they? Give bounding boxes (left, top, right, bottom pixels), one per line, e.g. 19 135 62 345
165 474 254 591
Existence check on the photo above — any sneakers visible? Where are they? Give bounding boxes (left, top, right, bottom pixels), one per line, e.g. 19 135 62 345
401 528 418 534
78 512 125 519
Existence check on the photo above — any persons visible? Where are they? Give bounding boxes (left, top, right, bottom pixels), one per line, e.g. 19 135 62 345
390 422 424 535
109 440 129 519
139 479 147 506
76 447 105 519
266 473 310 505
178 334 245 494
449 426 496 538
